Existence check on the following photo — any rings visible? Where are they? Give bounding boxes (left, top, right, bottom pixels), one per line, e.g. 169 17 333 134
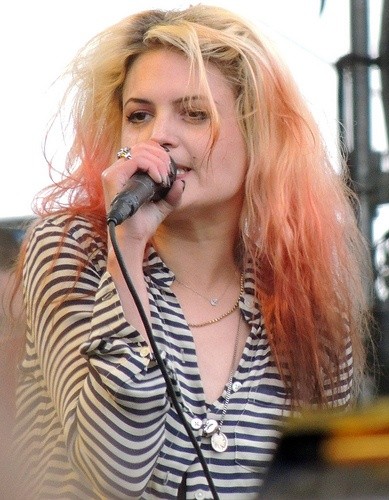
112 145 136 167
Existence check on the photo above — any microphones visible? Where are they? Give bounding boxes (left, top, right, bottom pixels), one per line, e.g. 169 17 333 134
107 146 177 226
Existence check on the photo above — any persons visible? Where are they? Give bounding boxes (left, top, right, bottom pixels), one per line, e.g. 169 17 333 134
9 1 375 500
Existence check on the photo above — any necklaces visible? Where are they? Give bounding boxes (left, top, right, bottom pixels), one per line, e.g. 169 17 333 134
157 247 244 456
167 258 252 333
149 242 246 308
